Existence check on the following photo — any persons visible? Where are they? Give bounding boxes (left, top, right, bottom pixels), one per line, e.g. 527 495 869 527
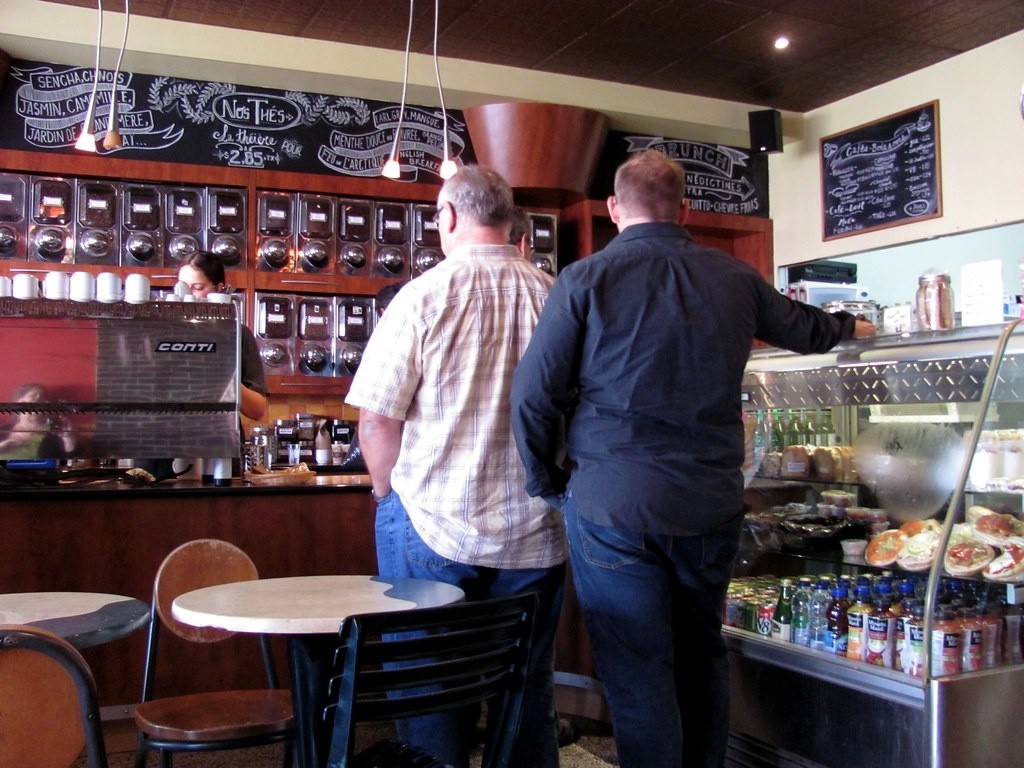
344 164 575 768
133 250 267 478
0 383 76 461
510 150 877 768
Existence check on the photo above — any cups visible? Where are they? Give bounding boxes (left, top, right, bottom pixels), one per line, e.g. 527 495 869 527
1 271 232 303
331 444 342 465
288 444 300 466
342 444 351 463
316 449 331 466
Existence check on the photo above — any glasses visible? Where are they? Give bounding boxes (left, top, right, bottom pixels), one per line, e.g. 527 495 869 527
430 204 447 226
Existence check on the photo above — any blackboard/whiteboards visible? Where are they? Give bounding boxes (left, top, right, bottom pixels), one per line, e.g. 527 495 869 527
0 49 772 220
817 98 944 242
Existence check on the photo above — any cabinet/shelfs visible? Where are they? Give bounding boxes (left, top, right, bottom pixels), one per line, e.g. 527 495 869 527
720 318 1024 768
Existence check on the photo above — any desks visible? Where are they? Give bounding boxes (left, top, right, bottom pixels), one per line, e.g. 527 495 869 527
172 575 468 768
0 591 151 650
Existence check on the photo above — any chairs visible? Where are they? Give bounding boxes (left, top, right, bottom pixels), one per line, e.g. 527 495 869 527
0 624 109 768
133 538 294 768
322 591 540 768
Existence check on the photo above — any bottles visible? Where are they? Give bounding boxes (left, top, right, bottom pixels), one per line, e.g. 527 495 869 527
342 419 354 443
331 419 342 444
243 441 253 476
742 409 838 478
770 571 1024 679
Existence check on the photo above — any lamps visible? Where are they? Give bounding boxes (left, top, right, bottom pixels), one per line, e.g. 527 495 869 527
75 0 131 153
433 0 457 179
382 0 414 179
